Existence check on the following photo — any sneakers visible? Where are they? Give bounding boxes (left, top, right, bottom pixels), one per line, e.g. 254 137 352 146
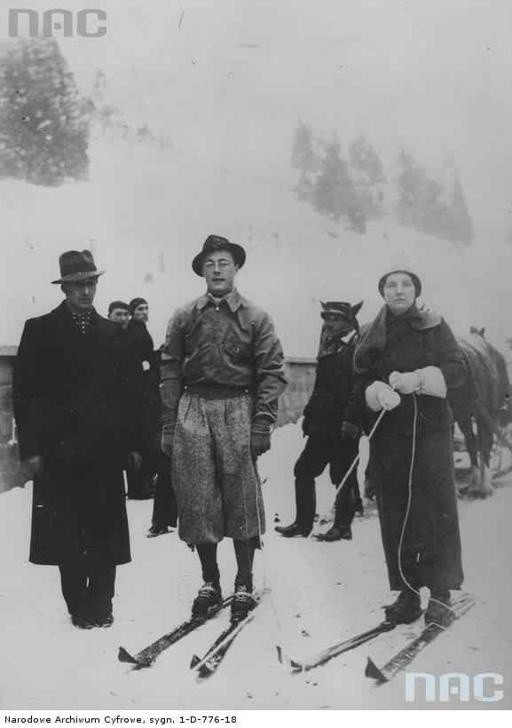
425 595 452 627
192 580 221 617
231 583 253 611
386 589 421 622
143 523 168 536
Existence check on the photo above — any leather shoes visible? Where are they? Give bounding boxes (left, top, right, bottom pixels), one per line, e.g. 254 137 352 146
312 522 351 542
274 522 313 537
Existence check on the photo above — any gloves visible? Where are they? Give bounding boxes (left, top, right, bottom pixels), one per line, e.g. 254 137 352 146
251 419 270 462
340 421 360 443
161 422 175 458
364 380 401 411
389 365 447 400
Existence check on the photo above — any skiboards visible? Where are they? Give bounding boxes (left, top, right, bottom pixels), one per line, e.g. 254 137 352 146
276 589 474 684
119 589 255 675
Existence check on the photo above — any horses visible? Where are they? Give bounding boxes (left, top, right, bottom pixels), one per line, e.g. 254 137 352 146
449 341 512 500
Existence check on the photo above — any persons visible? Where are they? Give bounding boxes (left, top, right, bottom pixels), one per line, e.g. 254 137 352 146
356 270 465 621
14 249 132 628
275 302 364 541
106 297 178 536
163 236 287 613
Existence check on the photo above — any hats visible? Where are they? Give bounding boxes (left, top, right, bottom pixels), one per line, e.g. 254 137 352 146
378 266 421 297
192 235 246 276
51 250 106 284
321 302 353 321
